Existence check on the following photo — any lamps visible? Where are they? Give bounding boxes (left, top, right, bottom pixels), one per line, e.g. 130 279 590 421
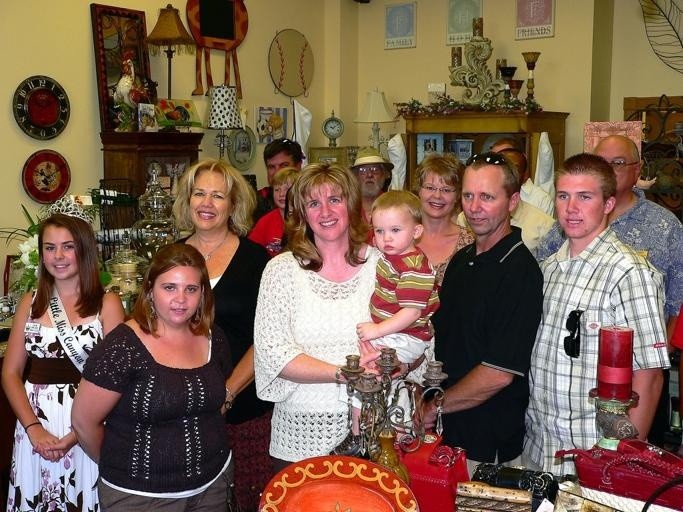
204 82 245 166
142 2 196 99
350 86 399 155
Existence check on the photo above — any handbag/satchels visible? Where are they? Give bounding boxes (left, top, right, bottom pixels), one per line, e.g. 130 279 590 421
471 462 556 510
393 433 470 512
557 435 683 511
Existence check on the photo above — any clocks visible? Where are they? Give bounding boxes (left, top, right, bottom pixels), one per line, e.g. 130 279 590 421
19 149 74 206
321 108 346 147
11 74 73 142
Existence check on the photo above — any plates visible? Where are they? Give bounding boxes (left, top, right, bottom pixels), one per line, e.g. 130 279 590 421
258 456 418 512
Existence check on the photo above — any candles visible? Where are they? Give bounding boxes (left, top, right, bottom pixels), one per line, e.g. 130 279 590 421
597 325 634 400
642 112 647 139
495 58 508 81
471 15 484 37
451 45 462 66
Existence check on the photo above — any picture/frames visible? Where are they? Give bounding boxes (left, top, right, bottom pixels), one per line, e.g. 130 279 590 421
90 2 155 130
226 124 258 172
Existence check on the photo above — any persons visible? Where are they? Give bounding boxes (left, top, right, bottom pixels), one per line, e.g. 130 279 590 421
1 134 682 512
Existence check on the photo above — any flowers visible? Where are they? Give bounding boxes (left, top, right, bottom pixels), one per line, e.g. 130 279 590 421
0 201 112 298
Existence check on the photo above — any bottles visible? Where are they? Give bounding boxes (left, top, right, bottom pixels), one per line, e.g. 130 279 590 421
105 169 174 315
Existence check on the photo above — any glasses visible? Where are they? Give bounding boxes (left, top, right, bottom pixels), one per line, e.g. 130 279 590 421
610 159 640 170
423 185 459 194
466 153 516 182
564 310 584 357
358 166 383 175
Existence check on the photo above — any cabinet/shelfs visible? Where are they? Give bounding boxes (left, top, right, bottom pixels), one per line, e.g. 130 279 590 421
399 108 570 194
309 146 360 167
100 130 206 237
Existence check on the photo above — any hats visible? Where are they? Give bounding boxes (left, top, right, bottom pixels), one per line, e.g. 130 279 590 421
348 146 394 171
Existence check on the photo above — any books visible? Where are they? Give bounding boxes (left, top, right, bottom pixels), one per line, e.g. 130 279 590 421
137 101 156 133
